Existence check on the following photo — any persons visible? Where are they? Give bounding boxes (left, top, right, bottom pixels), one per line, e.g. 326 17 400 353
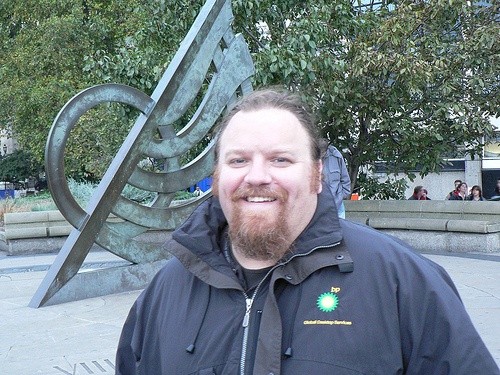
408 185 426 200
449 182 468 200
192 186 204 196
444 180 463 200
466 185 487 201
316 134 350 220
422 189 431 200
114 85 500 375
496 178 500 197
